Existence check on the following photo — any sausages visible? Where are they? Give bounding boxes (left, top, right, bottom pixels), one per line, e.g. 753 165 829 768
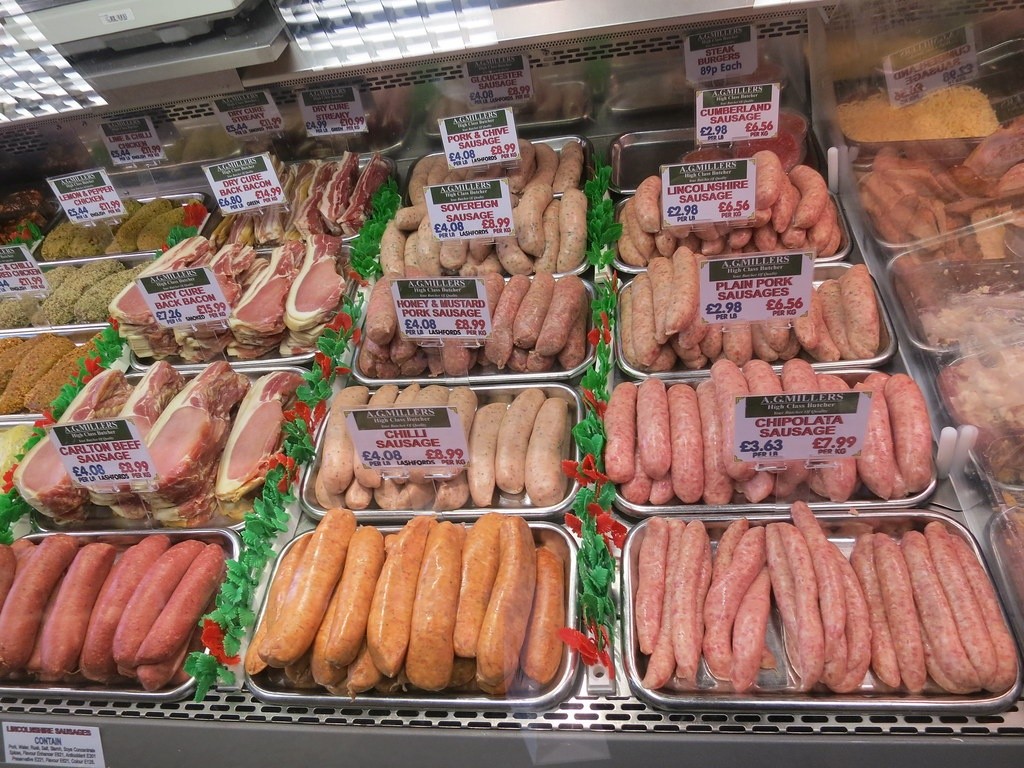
244 138 1020 698
0 532 229 693
